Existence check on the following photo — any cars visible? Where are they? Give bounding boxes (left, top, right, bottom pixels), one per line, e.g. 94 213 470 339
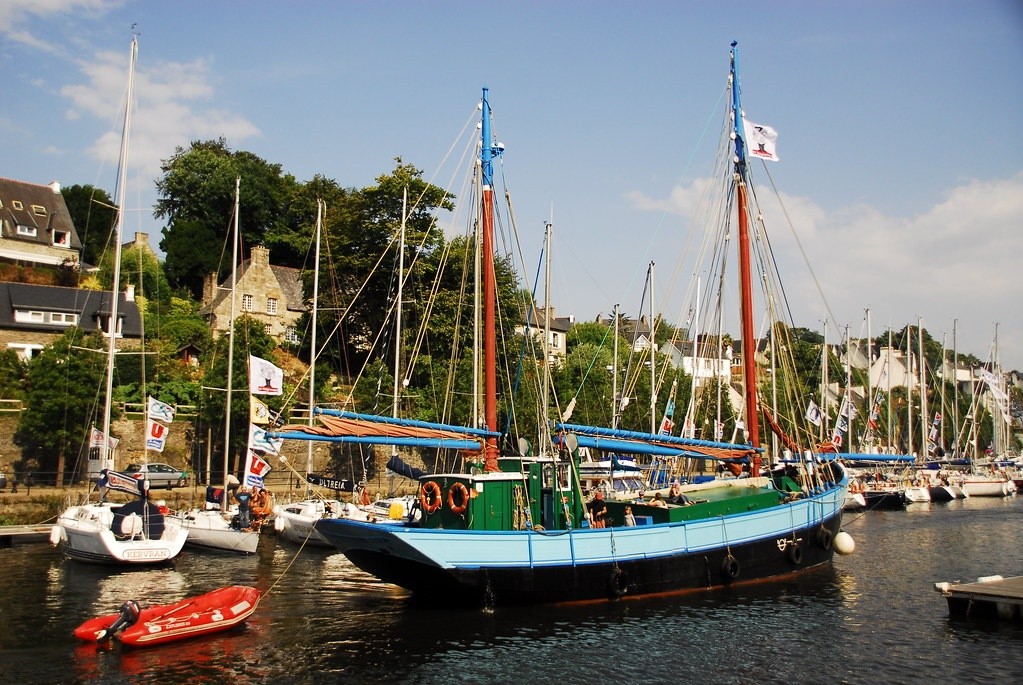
119 463 187 488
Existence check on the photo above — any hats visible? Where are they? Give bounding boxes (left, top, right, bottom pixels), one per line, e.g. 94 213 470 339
596 492 603 500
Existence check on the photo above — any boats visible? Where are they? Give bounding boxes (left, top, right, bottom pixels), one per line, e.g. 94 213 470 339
73 584 260 647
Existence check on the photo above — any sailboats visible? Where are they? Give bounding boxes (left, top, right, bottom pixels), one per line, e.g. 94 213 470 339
167 174 266 556
263 40 867 610
270 197 426 553
47 25 193 562
362 183 1023 514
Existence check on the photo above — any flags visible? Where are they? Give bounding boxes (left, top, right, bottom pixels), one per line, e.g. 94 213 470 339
661 401 724 439
804 400 825 426
928 412 941 452
246 354 283 491
743 117 780 162
832 397 857 446
146 396 174 453
867 392 885 431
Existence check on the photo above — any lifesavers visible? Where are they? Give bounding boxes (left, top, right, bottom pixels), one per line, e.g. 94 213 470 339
851 485 857 493
609 569 629 595
789 545 803 564
359 489 367 505
819 531 830 551
421 481 441 512
447 482 469 513
721 557 741 578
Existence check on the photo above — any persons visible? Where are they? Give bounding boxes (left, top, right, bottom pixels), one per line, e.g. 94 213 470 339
630 491 645 505
227 474 273 532
589 492 607 528
646 493 668 509
668 483 693 505
624 506 637 526
